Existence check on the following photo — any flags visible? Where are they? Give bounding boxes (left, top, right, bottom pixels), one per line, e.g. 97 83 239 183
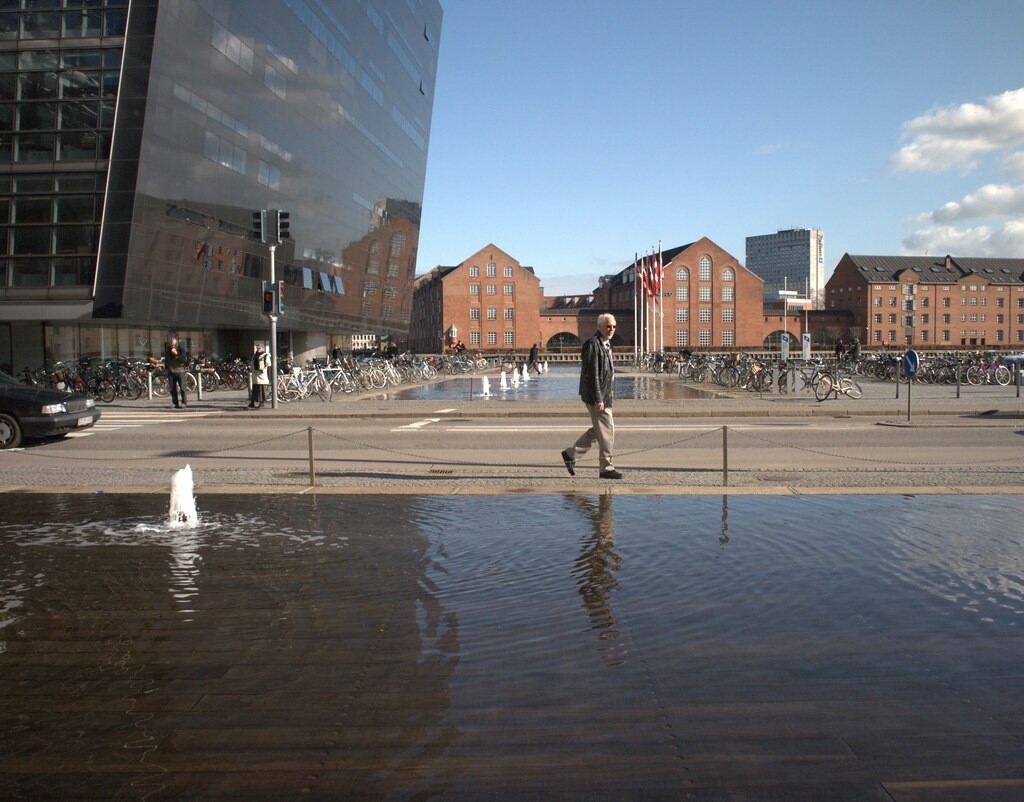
635 250 664 304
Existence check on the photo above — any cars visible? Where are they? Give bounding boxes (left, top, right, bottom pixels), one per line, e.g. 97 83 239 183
0 372 102 450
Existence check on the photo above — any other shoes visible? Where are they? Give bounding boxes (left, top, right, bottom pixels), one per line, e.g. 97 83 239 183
258 403 265 408
171 403 179 408
249 404 255 409
180 403 186 408
599 469 623 478
561 451 576 476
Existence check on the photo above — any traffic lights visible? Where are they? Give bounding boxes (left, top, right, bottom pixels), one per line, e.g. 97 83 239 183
264 290 272 314
279 280 285 314
252 208 266 242
277 211 290 245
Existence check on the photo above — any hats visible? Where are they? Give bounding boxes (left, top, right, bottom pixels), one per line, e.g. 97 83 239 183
256 343 264 349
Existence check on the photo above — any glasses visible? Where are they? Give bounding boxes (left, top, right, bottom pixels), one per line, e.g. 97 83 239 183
607 325 616 328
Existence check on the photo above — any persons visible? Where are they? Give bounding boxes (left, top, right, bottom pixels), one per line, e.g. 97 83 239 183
333 346 346 359
561 313 623 479
386 342 400 359
852 338 861 357
165 337 189 409
248 343 271 409
455 341 466 353
835 340 845 355
527 344 543 375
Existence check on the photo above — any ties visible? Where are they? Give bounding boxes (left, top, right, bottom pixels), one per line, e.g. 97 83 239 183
606 343 614 383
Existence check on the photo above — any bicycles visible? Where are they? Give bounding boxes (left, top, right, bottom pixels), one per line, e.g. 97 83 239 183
9 352 254 402
632 348 863 405
836 346 1024 389
278 348 487 403
